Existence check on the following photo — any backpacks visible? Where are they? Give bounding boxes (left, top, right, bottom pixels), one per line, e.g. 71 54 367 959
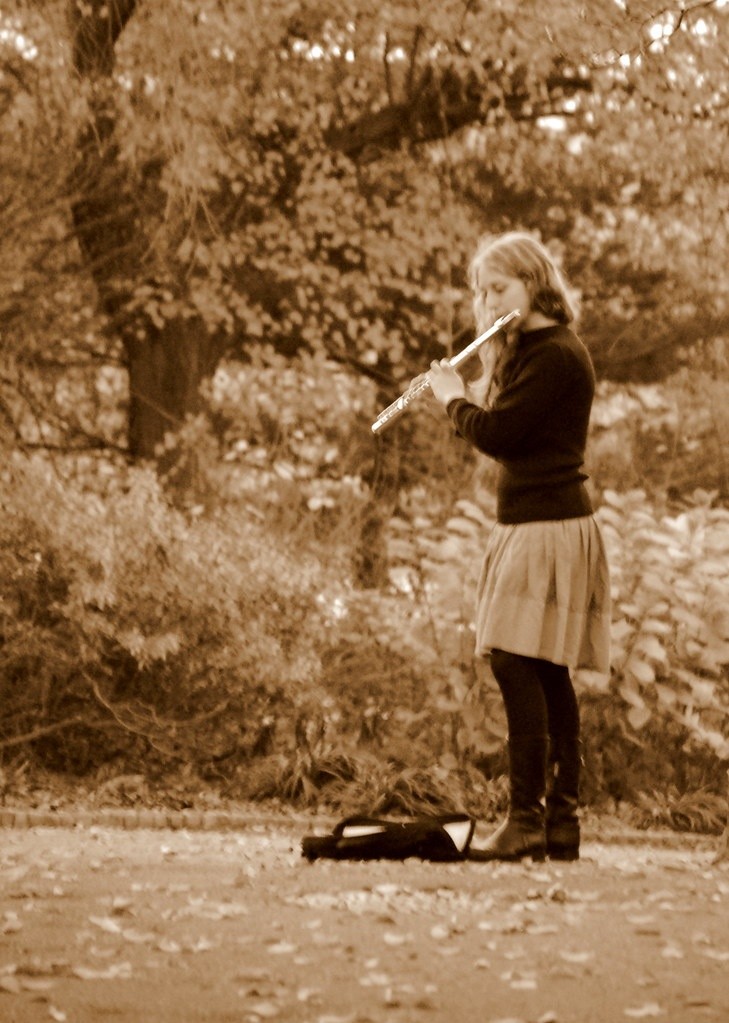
300 810 476 865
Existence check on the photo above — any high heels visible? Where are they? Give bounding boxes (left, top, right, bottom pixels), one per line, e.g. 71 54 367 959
546 814 581 861
462 812 548 863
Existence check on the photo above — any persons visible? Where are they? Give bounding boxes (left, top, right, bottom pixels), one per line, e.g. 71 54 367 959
406 227 614 864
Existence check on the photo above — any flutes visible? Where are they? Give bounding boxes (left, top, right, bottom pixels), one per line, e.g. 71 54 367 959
371 309 524 437
